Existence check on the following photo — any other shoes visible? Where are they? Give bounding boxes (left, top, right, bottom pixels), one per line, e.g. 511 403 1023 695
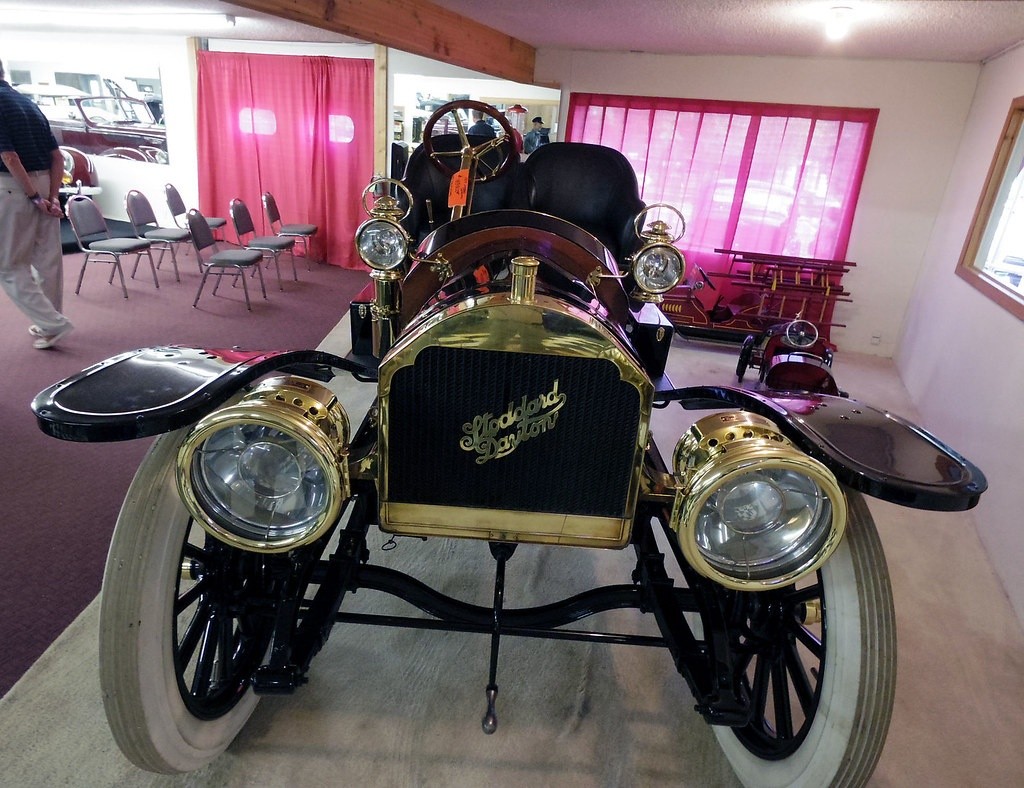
29 325 40 337
33 325 72 348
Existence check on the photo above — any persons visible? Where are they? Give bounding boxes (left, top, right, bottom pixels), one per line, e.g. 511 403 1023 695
0 56 74 353
468 111 495 140
524 116 544 153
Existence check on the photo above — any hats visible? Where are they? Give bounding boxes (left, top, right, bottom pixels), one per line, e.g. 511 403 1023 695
532 117 544 124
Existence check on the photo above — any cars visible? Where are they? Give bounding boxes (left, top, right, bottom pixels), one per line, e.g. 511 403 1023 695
53 139 104 224
40 93 171 165
27 99 989 787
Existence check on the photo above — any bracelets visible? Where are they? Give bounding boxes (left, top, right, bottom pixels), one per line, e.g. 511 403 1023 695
28 193 40 200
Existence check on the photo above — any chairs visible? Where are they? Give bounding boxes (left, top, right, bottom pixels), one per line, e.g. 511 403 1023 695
517 141 647 299
262 190 320 273
163 182 228 263
228 197 297 293
124 189 203 283
395 134 520 293
65 194 160 301
185 208 266 313
